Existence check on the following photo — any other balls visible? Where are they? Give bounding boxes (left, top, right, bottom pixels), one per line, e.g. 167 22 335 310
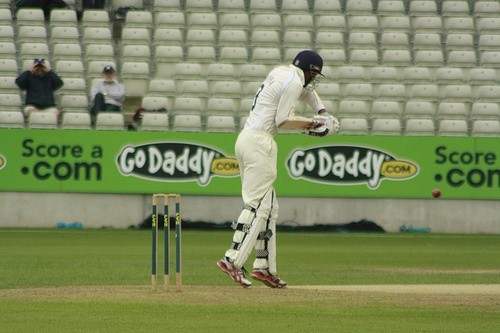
432 189 441 199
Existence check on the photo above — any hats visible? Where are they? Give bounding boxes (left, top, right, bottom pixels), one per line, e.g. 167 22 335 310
104 67 114 72
34 58 45 67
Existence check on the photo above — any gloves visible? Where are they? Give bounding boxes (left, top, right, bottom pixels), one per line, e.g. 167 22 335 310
307 113 340 136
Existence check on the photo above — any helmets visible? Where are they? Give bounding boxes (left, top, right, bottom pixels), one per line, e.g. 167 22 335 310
292 50 326 78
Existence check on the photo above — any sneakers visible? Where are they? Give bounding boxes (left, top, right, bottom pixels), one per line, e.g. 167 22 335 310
216 257 252 288
250 270 287 289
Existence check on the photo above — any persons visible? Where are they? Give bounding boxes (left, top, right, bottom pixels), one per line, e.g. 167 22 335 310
91 66 127 114
215 49 340 288
15 57 64 109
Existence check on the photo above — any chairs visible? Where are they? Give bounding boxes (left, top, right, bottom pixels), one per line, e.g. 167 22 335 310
0 0 500 135
